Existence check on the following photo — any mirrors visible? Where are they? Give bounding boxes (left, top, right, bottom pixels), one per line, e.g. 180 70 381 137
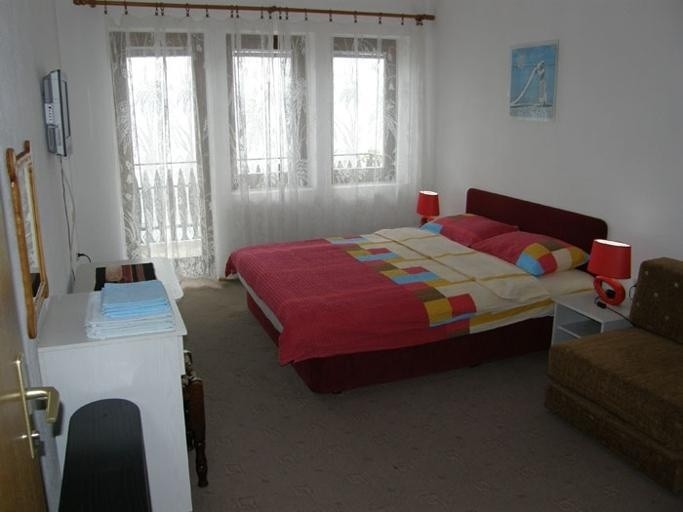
7 139 50 339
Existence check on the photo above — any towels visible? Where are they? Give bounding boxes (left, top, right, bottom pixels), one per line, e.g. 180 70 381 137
84 280 176 340
94 262 156 290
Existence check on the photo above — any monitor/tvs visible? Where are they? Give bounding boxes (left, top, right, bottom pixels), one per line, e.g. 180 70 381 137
42 69 74 160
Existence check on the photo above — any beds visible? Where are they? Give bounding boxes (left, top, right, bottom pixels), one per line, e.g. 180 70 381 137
224 187 608 395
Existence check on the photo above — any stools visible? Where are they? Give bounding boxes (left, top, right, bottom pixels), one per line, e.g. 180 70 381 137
180 348 210 487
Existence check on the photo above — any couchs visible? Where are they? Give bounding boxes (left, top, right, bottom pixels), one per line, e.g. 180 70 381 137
546 256 683 502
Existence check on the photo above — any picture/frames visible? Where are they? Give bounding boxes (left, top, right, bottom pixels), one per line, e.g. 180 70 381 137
506 39 559 121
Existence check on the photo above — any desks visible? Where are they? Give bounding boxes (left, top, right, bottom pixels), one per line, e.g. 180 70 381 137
36 289 194 511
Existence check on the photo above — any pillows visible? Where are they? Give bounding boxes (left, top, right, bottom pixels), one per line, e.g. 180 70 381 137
431 212 519 249
470 230 590 277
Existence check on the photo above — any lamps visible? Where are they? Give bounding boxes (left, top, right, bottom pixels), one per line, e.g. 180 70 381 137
416 190 440 228
588 239 632 305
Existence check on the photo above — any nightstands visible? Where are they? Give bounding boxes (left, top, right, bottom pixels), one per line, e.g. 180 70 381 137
550 289 631 347
